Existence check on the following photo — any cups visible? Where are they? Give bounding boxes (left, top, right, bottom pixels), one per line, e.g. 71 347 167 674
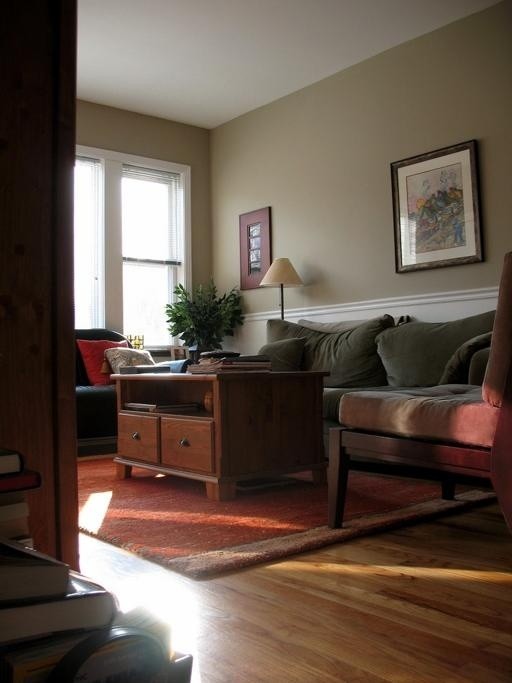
127 334 146 349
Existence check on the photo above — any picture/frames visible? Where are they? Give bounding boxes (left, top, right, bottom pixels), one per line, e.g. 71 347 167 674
390 139 484 274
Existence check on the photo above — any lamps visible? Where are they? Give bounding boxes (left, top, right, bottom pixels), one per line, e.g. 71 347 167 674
258 256 306 320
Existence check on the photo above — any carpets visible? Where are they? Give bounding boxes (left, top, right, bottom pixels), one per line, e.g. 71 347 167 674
78 452 495 579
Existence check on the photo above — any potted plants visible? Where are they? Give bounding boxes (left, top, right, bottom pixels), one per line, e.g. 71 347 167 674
165 282 245 362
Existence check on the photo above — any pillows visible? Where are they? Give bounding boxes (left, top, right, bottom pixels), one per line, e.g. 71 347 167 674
258 336 307 371
439 330 493 384
77 337 157 385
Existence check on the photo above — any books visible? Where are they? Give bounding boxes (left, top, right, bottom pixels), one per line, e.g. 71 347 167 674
1 543 174 682
187 352 273 373
2 450 43 550
236 477 296 492
123 395 201 414
120 364 171 374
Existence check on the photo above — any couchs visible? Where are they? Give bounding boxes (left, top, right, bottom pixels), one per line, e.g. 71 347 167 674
256 306 495 493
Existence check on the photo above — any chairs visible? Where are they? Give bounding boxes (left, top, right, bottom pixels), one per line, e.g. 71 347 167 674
75 327 199 458
326 249 512 532
